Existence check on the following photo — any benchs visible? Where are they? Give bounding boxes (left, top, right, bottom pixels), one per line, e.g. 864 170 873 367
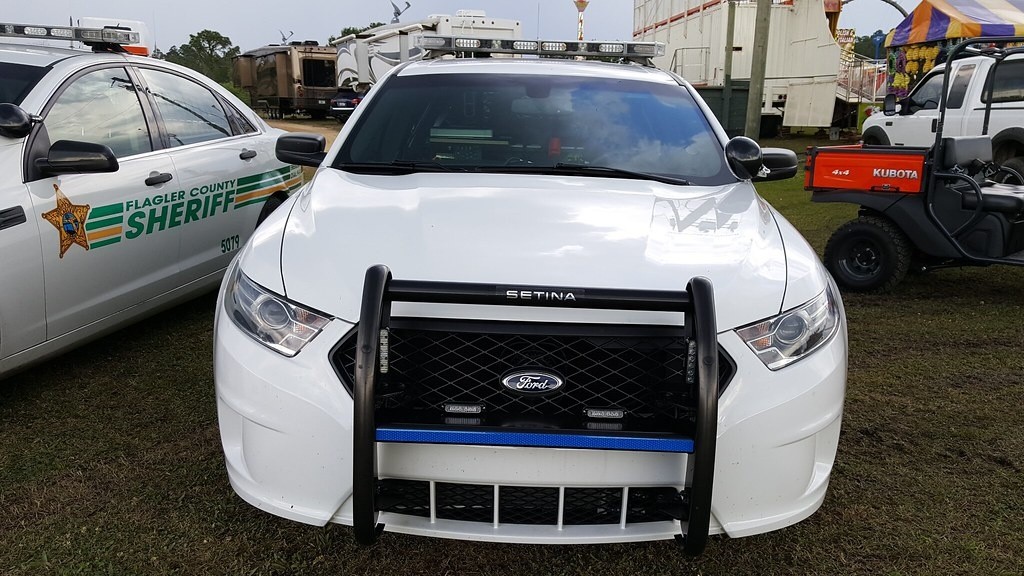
944 134 1024 216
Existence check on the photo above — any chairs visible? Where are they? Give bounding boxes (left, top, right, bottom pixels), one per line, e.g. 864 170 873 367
419 90 509 157
73 92 125 153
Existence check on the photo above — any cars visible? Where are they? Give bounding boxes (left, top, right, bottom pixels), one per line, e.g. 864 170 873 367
231 40 849 550
328 89 365 122
0 12 304 375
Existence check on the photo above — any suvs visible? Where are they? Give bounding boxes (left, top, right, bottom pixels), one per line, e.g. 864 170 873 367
863 52 1023 180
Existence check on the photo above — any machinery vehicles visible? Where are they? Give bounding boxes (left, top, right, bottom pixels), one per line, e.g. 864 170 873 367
232 0 525 120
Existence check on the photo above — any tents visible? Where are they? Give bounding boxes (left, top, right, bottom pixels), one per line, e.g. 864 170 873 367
884 0 1024 98
